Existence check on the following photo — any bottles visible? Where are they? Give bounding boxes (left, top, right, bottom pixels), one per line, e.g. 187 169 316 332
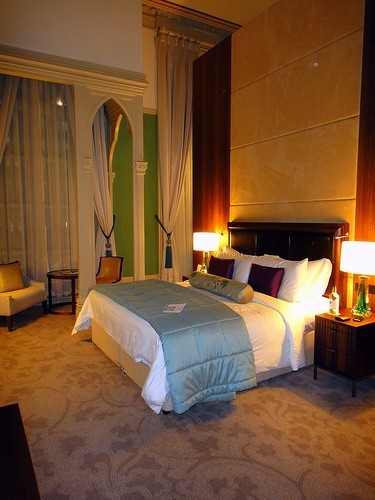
330 285 340 315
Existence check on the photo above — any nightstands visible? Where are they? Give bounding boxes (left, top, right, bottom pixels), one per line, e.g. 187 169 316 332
314 308 375 398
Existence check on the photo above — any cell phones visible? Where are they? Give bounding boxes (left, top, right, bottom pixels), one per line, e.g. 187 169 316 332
353 316 363 322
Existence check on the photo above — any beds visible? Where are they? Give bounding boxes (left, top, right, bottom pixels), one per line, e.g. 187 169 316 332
73 222 350 415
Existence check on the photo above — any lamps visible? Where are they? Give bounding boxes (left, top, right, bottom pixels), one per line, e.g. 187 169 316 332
193 232 220 271
339 241 375 318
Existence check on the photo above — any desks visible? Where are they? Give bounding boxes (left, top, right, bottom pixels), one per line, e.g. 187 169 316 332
47 269 78 315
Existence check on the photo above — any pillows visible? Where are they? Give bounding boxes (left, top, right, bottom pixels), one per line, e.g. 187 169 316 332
188 245 332 304
0 260 25 293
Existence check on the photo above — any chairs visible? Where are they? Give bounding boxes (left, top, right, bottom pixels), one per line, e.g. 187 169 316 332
95 256 123 284
0 261 47 330
